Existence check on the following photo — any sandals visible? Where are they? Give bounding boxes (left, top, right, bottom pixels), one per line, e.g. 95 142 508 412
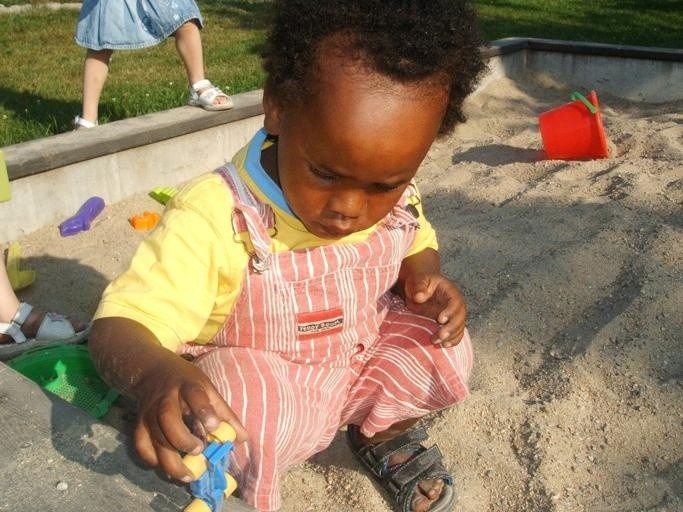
347 423 452 508
72 114 99 132
188 79 233 112
0 301 90 363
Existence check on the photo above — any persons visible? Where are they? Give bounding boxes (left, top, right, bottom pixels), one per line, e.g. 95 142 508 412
89 0 472 512
0 144 89 363
73 0 234 132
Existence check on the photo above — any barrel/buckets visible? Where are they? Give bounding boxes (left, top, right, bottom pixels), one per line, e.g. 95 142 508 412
538 90 609 159
